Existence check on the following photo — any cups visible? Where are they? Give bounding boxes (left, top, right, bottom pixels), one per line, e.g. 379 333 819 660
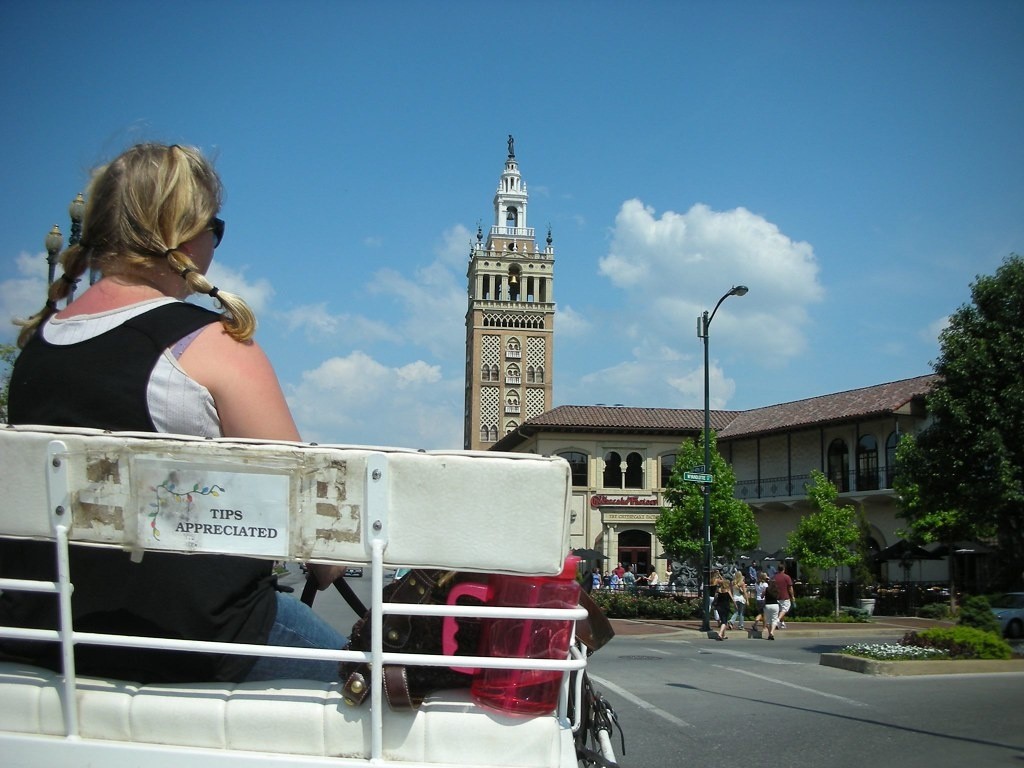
442 553 581 714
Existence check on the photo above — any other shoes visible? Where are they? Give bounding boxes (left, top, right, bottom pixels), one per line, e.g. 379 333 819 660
739 625 744 630
781 624 788 629
723 637 728 639
752 625 757 631
776 624 779 629
770 634 774 640
728 621 732 629
716 634 722 641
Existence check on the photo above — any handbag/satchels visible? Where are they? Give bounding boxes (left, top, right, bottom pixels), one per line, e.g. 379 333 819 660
341 566 615 713
739 586 744 595
714 609 720 621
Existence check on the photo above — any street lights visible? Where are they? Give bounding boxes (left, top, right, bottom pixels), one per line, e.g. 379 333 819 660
45 192 98 306
692 285 749 632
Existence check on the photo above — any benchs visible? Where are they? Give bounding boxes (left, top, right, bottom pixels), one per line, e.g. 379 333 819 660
0 424 590 768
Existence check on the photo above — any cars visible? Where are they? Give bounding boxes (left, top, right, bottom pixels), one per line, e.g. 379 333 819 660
987 593 1024 639
394 568 411 580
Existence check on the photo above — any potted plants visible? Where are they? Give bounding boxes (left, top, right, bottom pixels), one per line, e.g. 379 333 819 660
855 589 876 617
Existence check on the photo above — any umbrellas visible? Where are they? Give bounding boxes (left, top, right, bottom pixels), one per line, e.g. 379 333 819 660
871 539 945 580
933 542 963 559
568 549 610 577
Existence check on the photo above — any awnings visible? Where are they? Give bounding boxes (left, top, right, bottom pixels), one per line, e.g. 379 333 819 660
956 544 989 565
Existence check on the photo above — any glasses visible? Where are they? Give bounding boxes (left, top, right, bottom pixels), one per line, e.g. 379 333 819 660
202 217 225 248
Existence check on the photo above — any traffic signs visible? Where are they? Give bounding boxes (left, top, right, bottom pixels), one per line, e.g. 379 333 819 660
683 472 713 483
694 465 705 473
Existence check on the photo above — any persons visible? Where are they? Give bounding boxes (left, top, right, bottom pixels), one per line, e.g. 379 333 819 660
610 570 619 590
749 562 758 586
1 142 351 684
614 562 625 590
765 564 777 579
752 571 770 631
727 570 749 630
761 578 779 640
592 568 602 589
643 564 658 590
712 580 738 641
794 576 996 618
774 564 795 629
708 571 723 628
602 571 611 589
668 560 736 592
664 565 672 583
622 563 641 590
